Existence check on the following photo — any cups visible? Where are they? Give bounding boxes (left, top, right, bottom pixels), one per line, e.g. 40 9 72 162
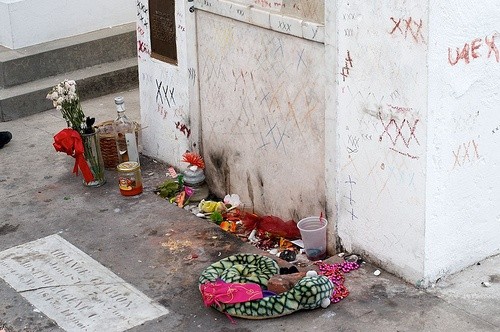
296 216 328 262
117 161 143 196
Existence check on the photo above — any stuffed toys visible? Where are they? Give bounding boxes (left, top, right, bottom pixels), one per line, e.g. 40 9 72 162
198 252 337 325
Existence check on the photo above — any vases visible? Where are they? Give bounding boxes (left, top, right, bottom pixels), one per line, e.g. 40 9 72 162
183 168 209 202
74 126 107 188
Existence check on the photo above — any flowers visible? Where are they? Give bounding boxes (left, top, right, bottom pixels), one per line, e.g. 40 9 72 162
45 78 103 182
180 151 206 169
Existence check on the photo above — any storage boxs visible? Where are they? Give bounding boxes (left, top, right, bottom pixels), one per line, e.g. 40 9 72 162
96 120 139 172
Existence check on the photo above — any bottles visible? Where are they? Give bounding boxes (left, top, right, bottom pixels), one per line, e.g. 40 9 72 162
113 95 140 166
